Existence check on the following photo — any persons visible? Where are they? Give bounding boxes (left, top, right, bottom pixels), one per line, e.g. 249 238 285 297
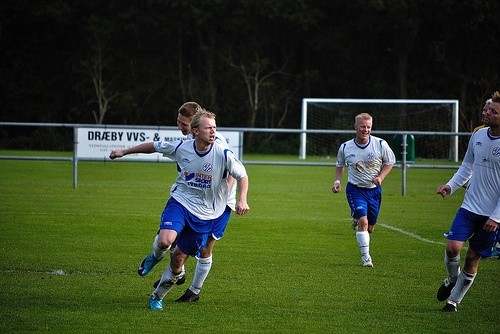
436 91 500 312
110 103 250 309
332 113 394 267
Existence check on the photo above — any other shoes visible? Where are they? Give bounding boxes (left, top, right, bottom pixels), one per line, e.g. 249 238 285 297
362 255 373 267
352 219 358 230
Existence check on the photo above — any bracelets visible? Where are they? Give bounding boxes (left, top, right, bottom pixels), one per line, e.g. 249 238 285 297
335 180 340 184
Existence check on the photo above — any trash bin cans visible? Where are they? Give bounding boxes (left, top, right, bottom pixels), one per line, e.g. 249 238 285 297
394 134 415 162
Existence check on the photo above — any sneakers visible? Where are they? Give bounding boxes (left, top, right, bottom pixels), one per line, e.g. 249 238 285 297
137 253 165 276
150 293 163 310
443 299 459 312
152 270 186 290
436 271 462 302
175 288 200 303
